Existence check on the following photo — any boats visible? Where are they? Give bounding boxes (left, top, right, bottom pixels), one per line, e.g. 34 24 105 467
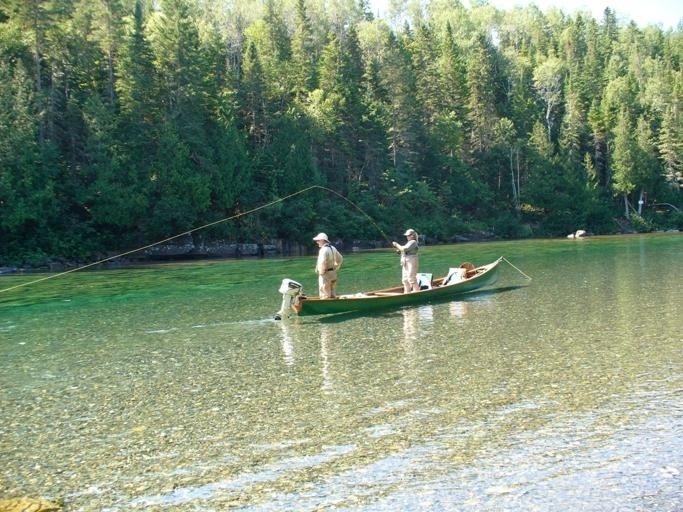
289 256 505 317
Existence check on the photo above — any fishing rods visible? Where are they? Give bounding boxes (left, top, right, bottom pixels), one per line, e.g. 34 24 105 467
312 186 401 256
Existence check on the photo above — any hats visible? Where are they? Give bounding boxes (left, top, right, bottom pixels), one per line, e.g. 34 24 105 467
403 228 415 236
313 233 328 241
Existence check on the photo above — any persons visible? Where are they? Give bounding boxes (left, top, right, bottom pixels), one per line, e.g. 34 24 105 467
312 233 343 300
392 228 420 293
